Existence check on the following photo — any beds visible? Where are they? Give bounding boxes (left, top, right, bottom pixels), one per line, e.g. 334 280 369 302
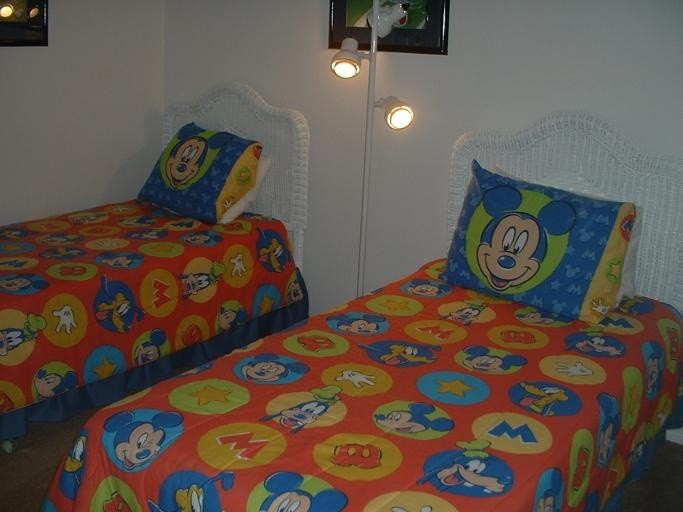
40 254 683 507
0 197 307 458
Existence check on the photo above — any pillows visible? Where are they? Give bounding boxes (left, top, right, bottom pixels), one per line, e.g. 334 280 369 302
136 121 271 225
436 156 636 326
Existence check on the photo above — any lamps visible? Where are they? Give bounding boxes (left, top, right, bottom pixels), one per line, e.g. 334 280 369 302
329 0 414 300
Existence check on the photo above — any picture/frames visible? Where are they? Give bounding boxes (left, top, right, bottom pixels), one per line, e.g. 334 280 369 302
0 0 50 51
329 0 450 56
